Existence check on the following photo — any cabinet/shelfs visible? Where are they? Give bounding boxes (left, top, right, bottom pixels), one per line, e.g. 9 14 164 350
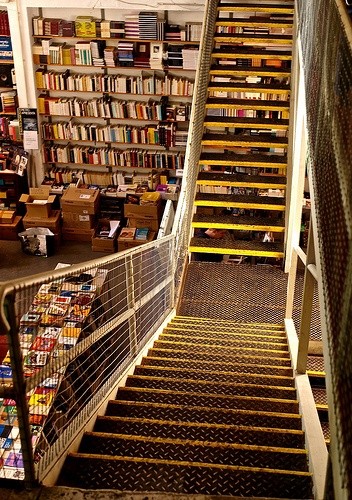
31 33 288 202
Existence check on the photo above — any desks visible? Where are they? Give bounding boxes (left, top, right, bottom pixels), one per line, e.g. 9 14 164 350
0 262 111 482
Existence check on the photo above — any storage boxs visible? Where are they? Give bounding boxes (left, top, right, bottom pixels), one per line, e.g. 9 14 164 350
0 188 159 253
